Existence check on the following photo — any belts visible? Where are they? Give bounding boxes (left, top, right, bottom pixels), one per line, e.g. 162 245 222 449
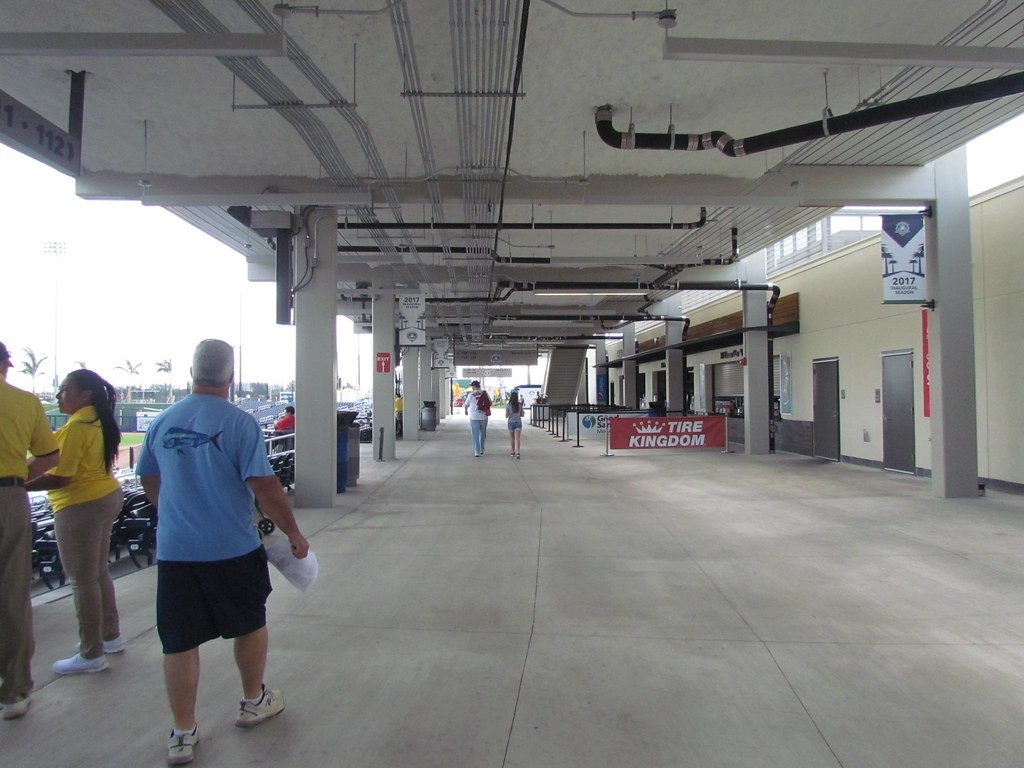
0 476 26 487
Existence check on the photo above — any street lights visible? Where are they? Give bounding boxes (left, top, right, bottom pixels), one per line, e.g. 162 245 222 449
41 240 67 406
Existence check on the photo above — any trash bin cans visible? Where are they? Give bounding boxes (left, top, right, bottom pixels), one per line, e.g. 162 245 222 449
336 411 361 493
421 401 437 432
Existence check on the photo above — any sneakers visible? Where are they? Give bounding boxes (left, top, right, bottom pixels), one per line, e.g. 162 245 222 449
236 683 286 726
76 635 125 655
166 721 199 763
52 651 107 674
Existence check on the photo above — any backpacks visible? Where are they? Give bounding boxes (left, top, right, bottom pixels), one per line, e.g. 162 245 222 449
477 392 490 411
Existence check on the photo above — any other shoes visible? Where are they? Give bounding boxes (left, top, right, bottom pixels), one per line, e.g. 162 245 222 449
2 698 30 719
517 453 520 458
480 451 483 455
510 452 516 457
475 453 479 457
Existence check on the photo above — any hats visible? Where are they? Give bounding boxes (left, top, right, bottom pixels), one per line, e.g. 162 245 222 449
0 341 13 367
470 381 480 387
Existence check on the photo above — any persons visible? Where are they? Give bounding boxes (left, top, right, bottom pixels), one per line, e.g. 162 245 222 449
464 380 492 457
0 342 59 720
505 392 525 459
135 339 311 766
394 392 403 420
274 406 294 451
24 369 128 677
